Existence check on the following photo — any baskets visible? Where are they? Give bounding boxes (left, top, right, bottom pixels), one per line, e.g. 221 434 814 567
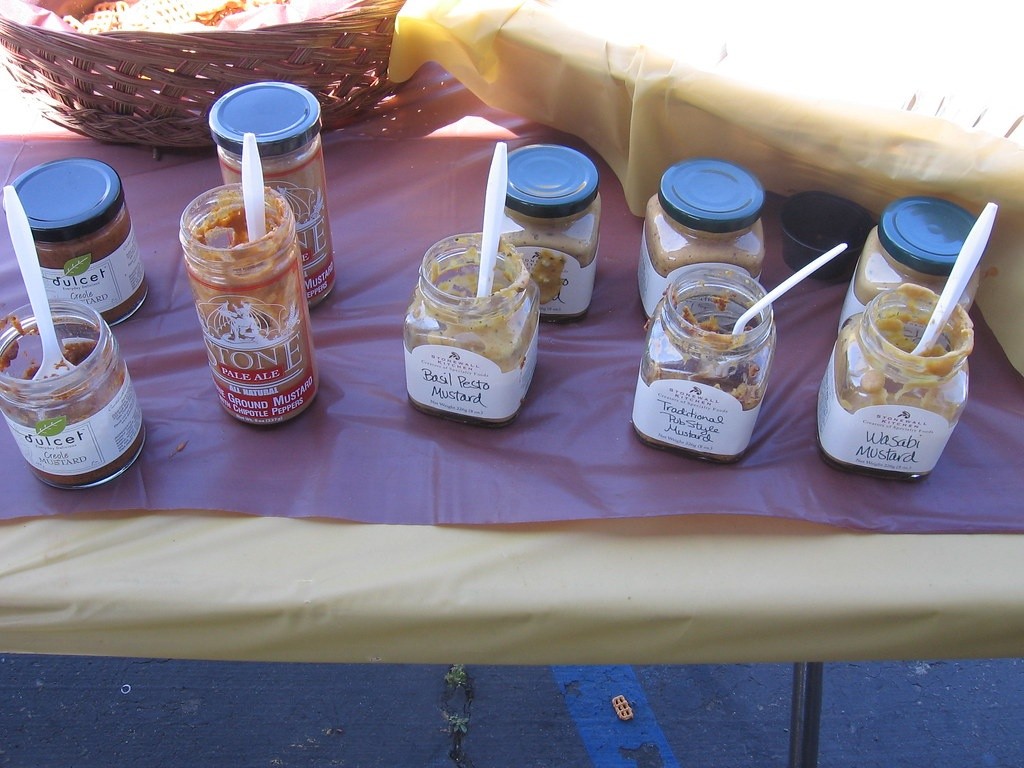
0 0 405 147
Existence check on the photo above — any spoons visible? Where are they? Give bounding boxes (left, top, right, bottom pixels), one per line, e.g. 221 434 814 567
2 184 84 389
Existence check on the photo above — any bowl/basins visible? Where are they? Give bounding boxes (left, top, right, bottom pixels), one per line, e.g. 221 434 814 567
779 190 877 282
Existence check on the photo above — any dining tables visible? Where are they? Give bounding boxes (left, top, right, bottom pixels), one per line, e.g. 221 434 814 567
0 0 1022 767
